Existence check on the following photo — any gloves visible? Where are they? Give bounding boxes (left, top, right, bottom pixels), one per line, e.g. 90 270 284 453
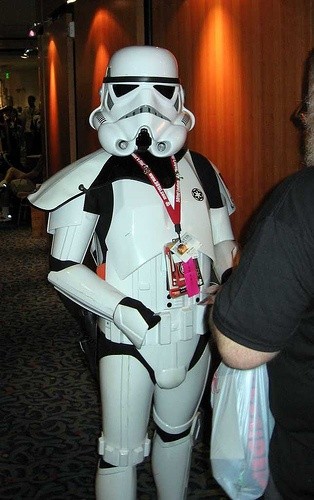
113 297 162 349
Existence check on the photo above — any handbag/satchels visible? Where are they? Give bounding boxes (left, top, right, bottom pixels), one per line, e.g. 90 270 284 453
211 359 275 500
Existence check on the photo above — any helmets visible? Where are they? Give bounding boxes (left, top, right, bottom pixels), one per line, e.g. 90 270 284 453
89 46 195 158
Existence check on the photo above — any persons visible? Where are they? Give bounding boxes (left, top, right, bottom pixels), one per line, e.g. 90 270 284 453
208 66 314 500
28 44 239 500
0 95 46 193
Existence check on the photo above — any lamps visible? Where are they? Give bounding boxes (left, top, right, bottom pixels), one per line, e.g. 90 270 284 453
20 48 37 59
29 22 41 37
